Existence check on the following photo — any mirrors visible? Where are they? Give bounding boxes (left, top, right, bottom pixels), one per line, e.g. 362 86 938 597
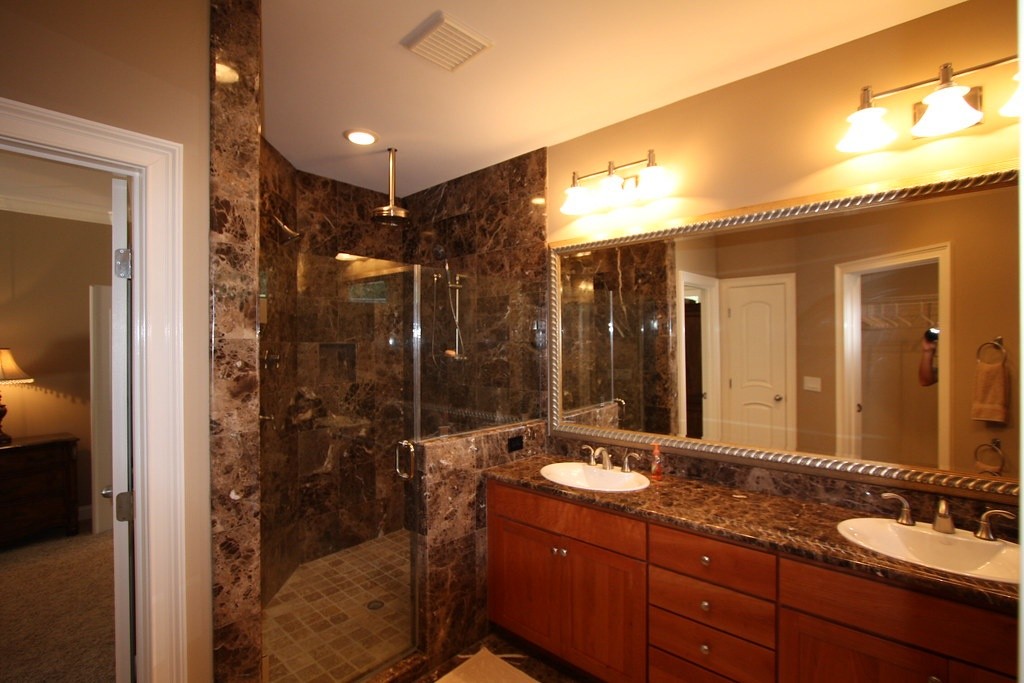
545 169 1019 503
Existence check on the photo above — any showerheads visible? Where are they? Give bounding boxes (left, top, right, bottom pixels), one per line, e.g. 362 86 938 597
433 244 451 283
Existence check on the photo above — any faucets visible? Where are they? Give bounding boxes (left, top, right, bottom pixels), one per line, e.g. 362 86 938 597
934 495 956 534
594 447 614 470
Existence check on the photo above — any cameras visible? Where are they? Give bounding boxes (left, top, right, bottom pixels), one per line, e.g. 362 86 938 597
925 328 940 341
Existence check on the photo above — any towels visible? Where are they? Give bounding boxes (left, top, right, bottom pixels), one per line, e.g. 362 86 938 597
973 361 1008 424
976 462 1001 477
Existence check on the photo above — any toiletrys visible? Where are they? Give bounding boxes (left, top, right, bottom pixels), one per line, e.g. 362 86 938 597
651 444 663 481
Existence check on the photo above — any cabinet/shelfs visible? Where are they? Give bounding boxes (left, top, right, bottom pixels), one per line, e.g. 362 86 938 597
0 432 78 551
482 481 1020 683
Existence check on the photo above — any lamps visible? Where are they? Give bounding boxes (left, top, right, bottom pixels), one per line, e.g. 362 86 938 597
561 150 667 215
0 348 35 445
838 56 1020 153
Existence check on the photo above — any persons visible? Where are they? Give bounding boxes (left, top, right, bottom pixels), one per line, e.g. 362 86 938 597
918 328 938 387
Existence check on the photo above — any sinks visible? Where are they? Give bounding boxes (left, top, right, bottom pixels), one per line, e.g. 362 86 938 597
540 462 650 492
838 516 1021 584
